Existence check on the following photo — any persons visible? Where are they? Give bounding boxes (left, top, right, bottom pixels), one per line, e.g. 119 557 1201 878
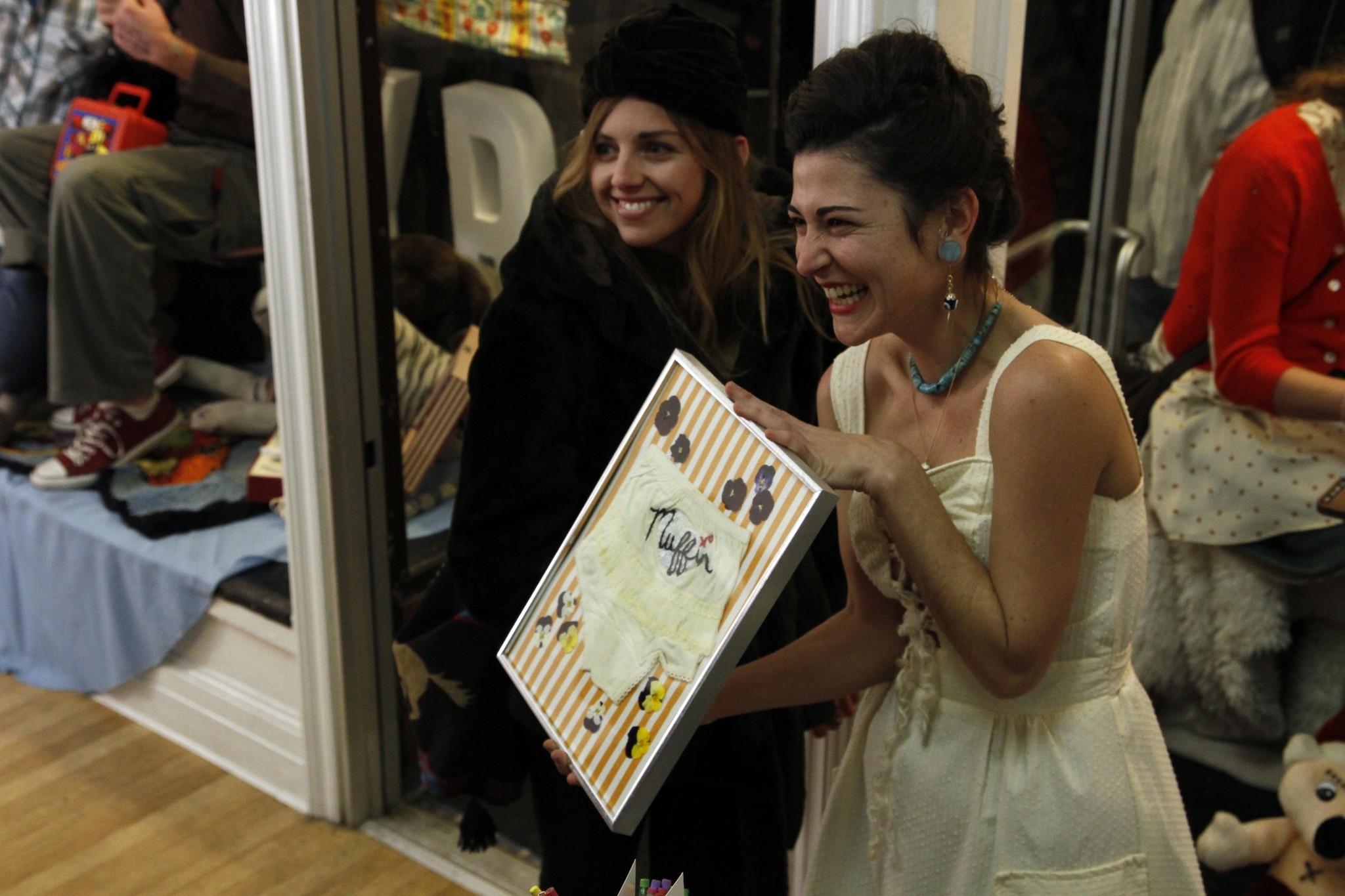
1117 68 1342 580
1 0 265 489
536 24 1212 896
436 3 853 896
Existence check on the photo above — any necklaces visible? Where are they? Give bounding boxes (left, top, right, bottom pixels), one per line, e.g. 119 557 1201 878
908 273 1004 473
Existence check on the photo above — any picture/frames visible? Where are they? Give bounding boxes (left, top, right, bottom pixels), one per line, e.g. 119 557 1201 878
495 350 842 838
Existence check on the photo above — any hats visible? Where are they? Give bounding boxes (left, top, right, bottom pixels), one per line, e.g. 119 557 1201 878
580 9 749 133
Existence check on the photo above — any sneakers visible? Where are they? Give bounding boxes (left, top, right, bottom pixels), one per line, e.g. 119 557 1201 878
29 390 182 490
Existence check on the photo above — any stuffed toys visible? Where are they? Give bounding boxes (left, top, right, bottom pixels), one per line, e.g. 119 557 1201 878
1196 703 1345 896
